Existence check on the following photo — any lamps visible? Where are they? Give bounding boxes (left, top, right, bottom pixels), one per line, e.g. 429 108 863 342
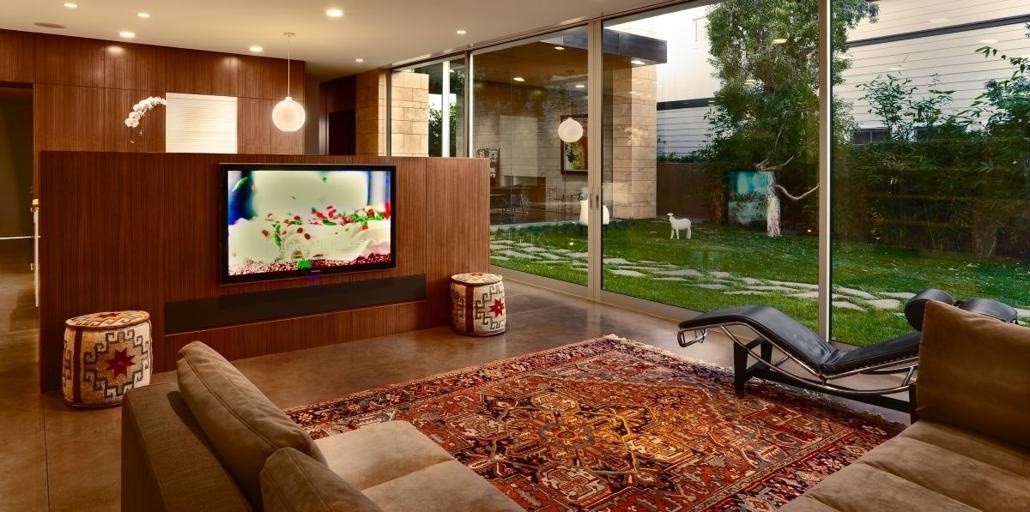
272 32 306 133
557 69 584 144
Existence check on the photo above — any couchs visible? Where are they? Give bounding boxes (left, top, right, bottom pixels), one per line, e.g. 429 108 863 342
770 286 1030 511
115 338 532 510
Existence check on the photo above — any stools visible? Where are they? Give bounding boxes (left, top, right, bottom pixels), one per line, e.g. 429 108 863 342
449 272 509 337
61 310 154 411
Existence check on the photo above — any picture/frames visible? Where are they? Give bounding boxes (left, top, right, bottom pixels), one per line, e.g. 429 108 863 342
559 112 589 176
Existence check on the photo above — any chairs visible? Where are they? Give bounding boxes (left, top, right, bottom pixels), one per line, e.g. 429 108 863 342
673 283 1021 430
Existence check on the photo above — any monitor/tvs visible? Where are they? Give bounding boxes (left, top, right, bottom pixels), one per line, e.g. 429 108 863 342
219 162 396 286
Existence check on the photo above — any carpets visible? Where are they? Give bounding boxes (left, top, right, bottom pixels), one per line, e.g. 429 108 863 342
273 332 910 511
